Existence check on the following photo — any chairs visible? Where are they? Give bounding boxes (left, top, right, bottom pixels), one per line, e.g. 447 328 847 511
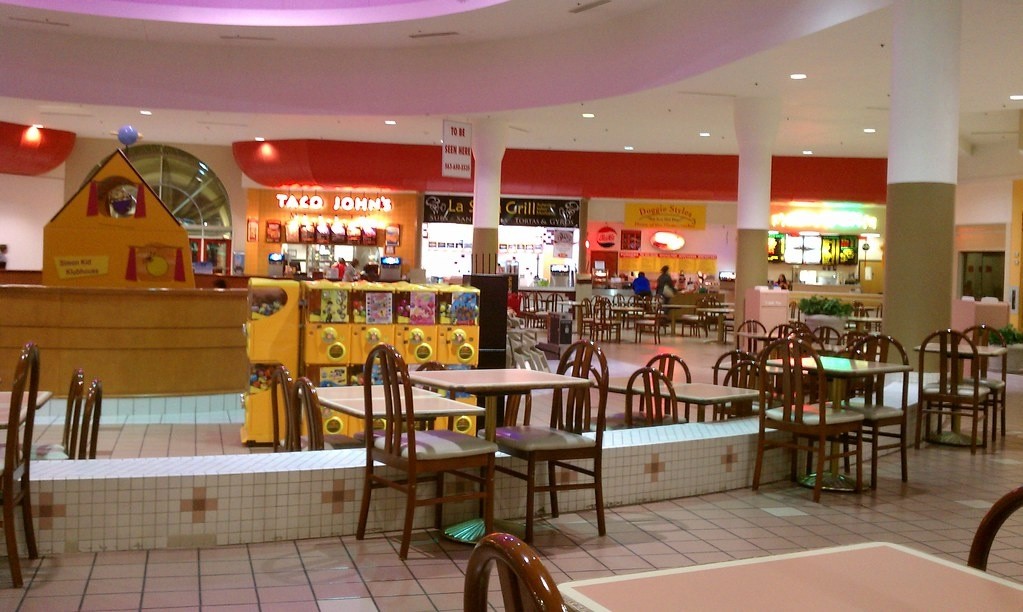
966 486 1023 584
354 341 499 561
0 341 42 588
290 376 325 450
477 338 607 535
26 366 84 462
462 531 567 612
522 284 1022 499
77 378 103 459
270 364 294 453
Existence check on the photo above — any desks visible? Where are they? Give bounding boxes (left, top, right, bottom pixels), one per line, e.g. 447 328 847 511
297 383 444 401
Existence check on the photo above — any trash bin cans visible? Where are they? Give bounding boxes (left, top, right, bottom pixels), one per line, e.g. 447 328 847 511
546 312 573 345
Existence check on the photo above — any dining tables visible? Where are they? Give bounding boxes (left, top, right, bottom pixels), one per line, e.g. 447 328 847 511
553 539 1023 612
392 367 594 545
331 396 486 449
0 390 53 428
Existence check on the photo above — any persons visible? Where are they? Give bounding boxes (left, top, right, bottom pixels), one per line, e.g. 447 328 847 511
342 258 361 282
655 265 673 327
331 258 346 279
776 274 789 290
631 271 652 297
